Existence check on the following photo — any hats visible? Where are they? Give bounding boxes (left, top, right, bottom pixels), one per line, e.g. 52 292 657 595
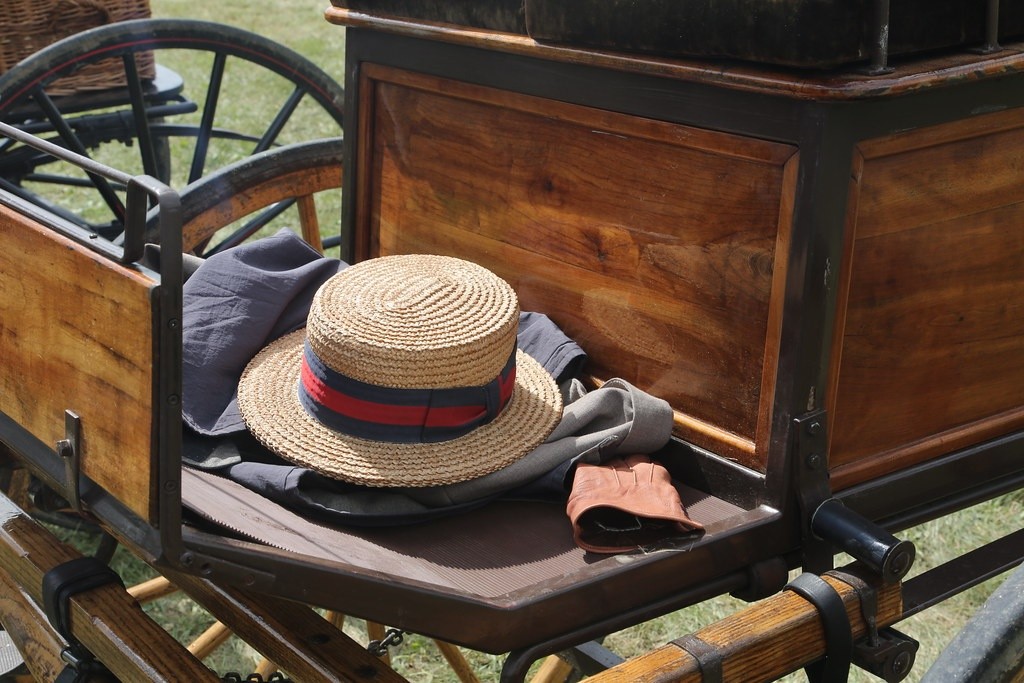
236 253 565 488
